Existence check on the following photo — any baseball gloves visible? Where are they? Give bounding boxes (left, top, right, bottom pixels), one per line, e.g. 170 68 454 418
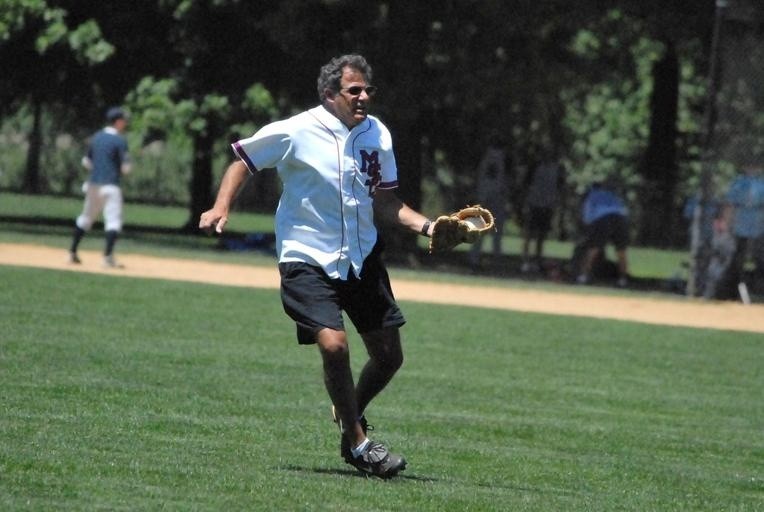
429 204 495 257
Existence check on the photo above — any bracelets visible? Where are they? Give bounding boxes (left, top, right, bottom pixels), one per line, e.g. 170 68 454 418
421 219 432 237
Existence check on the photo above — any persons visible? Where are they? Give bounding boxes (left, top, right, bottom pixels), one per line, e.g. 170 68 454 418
681 155 764 301
201 54 495 480
468 134 510 273
518 141 566 272
571 181 630 287
70 108 131 268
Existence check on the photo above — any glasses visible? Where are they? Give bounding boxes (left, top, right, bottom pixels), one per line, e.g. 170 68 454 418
341 85 378 96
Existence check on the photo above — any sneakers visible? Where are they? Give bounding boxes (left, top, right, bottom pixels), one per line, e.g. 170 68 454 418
471 251 628 289
340 433 408 481
332 400 374 439
103 255 124 270
68 250 80 263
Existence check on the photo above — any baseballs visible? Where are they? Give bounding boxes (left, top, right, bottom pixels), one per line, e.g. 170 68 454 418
463 215 488 231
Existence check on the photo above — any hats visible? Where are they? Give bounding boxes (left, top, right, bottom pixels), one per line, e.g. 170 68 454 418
107 109 128 121
